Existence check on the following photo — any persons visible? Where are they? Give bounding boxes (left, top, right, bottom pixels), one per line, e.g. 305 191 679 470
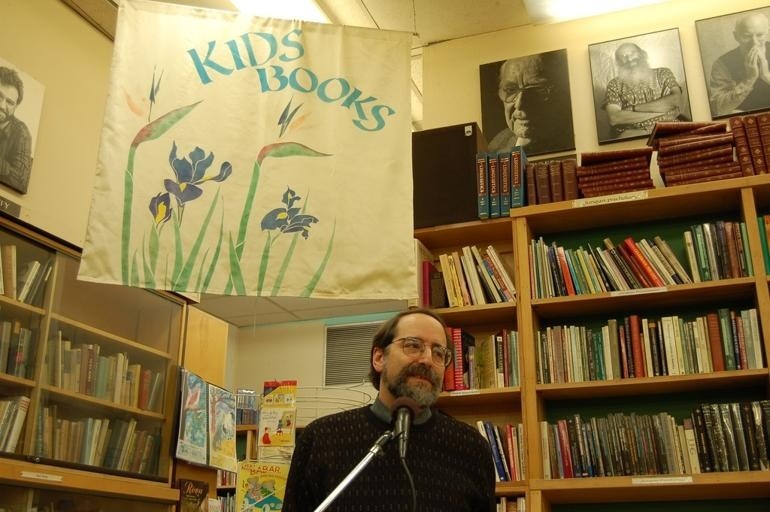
485 54 568 161
706 13 770 116
281 306 499 511
0 64 33 196
598 44 683 138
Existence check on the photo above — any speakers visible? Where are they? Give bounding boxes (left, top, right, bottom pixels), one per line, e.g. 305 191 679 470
410 122 490 228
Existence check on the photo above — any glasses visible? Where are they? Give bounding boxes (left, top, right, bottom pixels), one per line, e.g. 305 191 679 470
389 335 453 368
498 79 554 104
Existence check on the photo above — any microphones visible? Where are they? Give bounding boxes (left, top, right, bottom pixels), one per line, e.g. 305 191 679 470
392 396 419 459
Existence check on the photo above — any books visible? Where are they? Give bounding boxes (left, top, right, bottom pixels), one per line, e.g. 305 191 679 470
34 401 162 475
215 468 237 511
0 245 54 307
422 246 516 309
529 214 770 480
1 395 30 453
476 111 770 220
442 328 519 390
45 330 162 411
478 421 525 512
1 320 40 380
